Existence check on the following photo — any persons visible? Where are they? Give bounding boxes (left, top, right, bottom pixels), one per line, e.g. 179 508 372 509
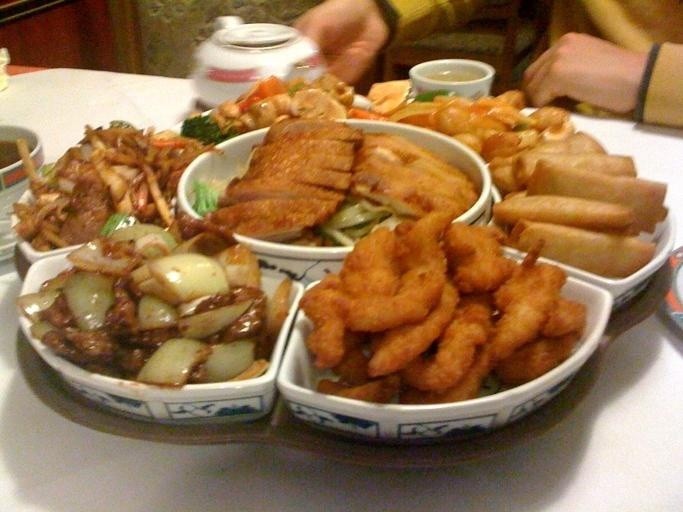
294 0 683 132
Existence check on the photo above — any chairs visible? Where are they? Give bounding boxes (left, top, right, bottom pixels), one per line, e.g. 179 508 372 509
381 1 551 94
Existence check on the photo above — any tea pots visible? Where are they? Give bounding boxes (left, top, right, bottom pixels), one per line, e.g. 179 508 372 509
189 14 328 110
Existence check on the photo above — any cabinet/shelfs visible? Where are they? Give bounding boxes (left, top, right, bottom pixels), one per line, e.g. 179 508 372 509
0 1 84 69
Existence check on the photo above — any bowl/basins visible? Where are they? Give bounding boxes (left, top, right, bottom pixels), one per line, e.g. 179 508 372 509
175 118 494 287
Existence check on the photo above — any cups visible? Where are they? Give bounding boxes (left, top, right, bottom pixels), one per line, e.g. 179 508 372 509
407 58 496 99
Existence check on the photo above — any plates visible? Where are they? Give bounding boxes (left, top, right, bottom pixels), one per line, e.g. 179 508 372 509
485 159 675 316
275 255 615 451
12 187 182 267
16 252 306 429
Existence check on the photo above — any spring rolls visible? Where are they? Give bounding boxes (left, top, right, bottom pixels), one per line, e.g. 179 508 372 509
488 132 668 279
